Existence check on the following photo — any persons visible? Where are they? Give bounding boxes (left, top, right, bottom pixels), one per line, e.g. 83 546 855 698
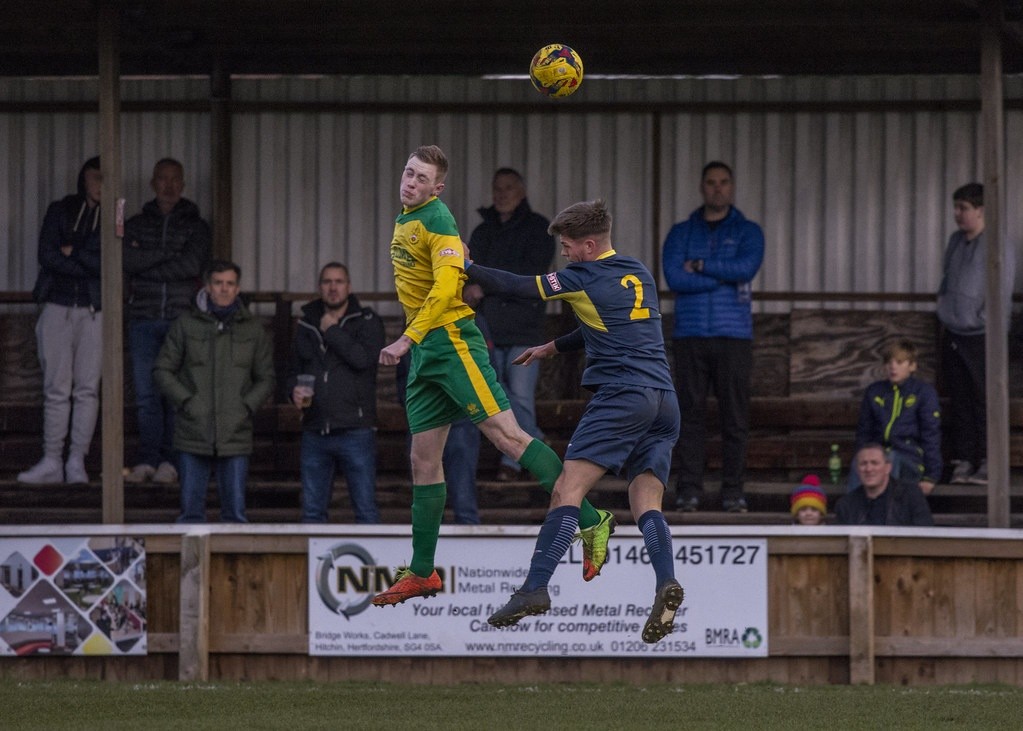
833 442 935 526
662 160 766 514
790 475 828 526
282 263 386 524
934 182 1015 486
371 145 618 608
395 168 555 526
461 201 685 644
854 337 944 495
122 157 210 488
151 261 276 523
17 155 104 485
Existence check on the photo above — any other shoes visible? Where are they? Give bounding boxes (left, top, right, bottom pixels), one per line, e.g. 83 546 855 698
676 488 699 512
497 465 520 481
720 490 748 513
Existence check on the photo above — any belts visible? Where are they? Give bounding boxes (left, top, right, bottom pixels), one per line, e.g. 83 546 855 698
47 296 93 307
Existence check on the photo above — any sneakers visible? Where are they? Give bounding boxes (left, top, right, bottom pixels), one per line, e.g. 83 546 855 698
66 458 89 483
572 509 615 581
642 577 684 644
126 463 155 482
18 457 64 484
152 461 177 483
488 588 551 629
968 459 988 485
370 559 442 608
949 460 971 483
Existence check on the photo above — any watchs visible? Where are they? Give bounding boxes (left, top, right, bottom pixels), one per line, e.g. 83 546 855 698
690 258 702 272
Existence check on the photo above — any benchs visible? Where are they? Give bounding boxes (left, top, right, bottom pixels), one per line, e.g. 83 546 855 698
0 289 1023 530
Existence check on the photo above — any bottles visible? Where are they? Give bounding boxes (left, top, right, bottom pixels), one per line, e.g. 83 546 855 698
827 445 844 486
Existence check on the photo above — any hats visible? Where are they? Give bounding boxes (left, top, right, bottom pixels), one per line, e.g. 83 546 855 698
790 475 826 517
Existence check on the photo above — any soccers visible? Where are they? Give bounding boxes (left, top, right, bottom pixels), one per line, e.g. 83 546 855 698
528 42 585 99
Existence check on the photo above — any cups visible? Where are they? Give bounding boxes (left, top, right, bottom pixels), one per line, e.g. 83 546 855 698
298 373 315 408
